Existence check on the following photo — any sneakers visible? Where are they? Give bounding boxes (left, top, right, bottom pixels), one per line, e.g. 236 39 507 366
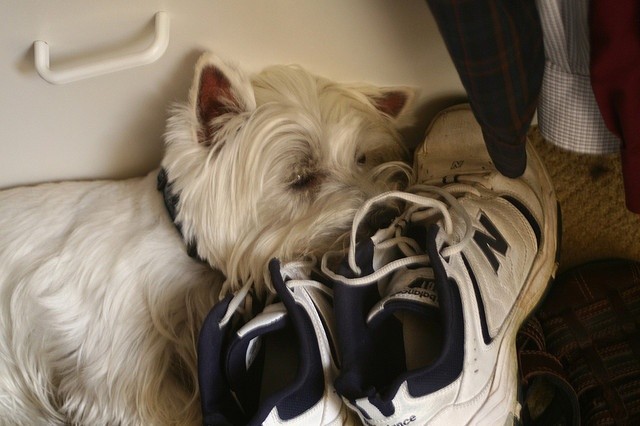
196 248 357 426
321 103 562 425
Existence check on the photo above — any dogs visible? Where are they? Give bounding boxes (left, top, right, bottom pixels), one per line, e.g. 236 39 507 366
1 49 417 426
